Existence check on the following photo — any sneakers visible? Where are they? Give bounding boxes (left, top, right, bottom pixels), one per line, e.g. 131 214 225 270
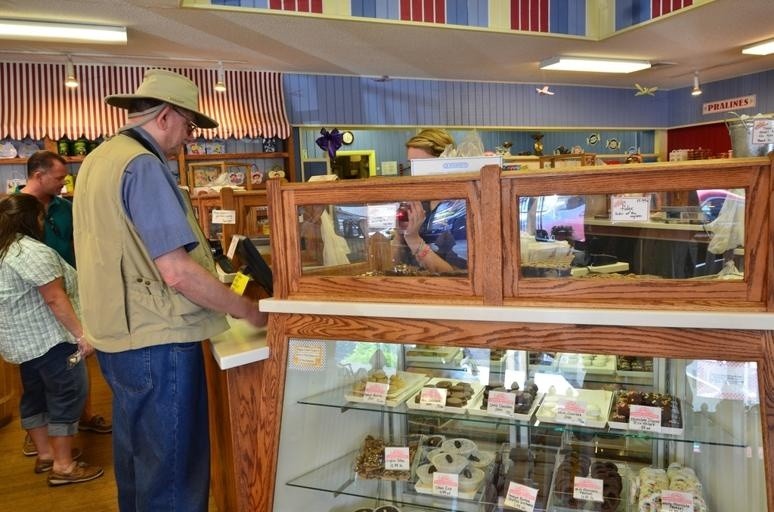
35 449 82 472
48 462 104 485
79 416 112 432
23 434 38 455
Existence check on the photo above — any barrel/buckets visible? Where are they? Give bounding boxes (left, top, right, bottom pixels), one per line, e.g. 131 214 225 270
723 111 769 156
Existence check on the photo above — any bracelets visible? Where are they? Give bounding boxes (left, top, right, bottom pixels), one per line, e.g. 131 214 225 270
75 335 85 342
411 237 424 256
415 245 431 262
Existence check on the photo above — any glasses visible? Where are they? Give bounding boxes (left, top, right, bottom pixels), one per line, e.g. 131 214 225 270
172 108 198 134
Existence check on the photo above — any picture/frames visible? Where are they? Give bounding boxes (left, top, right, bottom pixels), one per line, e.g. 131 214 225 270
188 161 225 195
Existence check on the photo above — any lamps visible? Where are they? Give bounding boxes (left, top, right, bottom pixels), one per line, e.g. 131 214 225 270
741 37 774 55
691 70 703 97
215 59 226 92
0 20 128 45
64 54 78 88
539 56 652 74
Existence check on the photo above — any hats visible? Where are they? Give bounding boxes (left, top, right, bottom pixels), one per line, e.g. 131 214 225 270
103 70 219 128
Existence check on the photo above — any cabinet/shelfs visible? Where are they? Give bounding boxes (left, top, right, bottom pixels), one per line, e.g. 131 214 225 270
297 124 665 187
189 189 325 512
1 126 294 220
248 151 773 511
582 224 732 281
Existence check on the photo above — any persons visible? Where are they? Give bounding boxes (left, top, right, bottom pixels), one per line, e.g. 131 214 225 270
7 150 113 456
390 126 469 273
616 153 662 209
71 69 269 512
0 192 104 484
